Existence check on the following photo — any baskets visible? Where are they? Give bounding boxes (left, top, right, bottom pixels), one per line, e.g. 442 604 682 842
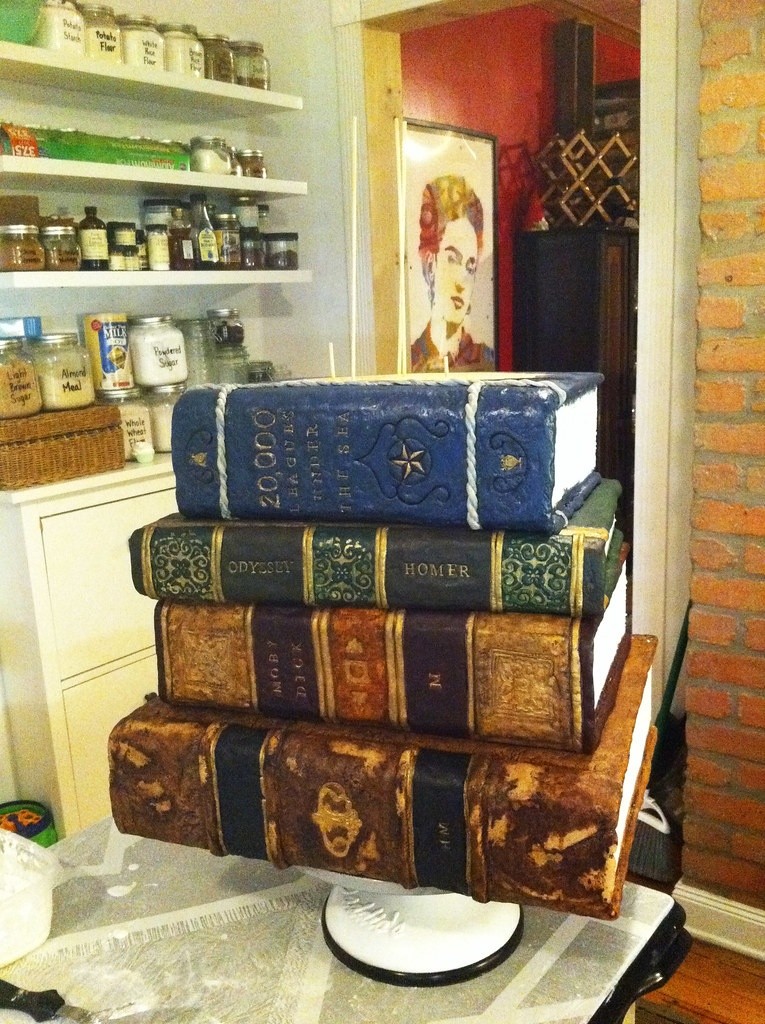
0 403 125 491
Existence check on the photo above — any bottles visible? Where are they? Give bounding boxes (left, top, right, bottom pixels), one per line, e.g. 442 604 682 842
190 194 220 271
258 205 272 270
168 207 195 271
77 206 110 271
199 203 225 271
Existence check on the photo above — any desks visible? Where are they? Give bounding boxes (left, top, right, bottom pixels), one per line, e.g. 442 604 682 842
0 815 673 1024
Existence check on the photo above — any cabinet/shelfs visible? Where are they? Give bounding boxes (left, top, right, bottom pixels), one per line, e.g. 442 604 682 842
0 38 313 288
0 471 179 840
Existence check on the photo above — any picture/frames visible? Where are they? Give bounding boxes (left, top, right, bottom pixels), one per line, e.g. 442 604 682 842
404 117 501 374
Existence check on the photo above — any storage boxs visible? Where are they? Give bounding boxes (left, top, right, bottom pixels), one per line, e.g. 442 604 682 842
0 122 191 172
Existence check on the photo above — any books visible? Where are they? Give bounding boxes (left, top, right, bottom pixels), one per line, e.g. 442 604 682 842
107 371 659 921
